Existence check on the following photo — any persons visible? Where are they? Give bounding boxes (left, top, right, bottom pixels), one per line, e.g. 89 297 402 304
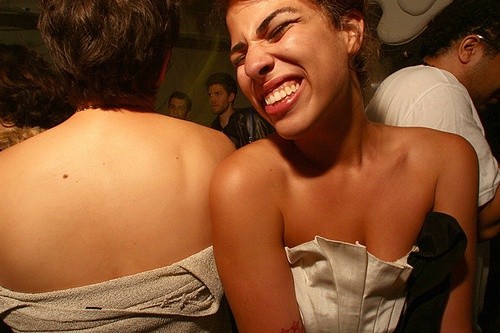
0 0 276 333
364 0 500 333
210 0 479 333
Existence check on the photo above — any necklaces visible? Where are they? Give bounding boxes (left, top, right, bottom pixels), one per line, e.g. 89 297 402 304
75 103 145 110
